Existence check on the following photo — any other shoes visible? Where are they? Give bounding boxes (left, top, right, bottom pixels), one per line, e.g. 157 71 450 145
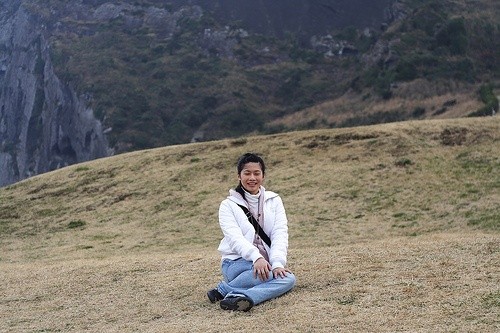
207 289 224 303
220 297 254 312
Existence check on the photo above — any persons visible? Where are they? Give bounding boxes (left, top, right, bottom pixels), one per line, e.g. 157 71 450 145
207 153 296 313
